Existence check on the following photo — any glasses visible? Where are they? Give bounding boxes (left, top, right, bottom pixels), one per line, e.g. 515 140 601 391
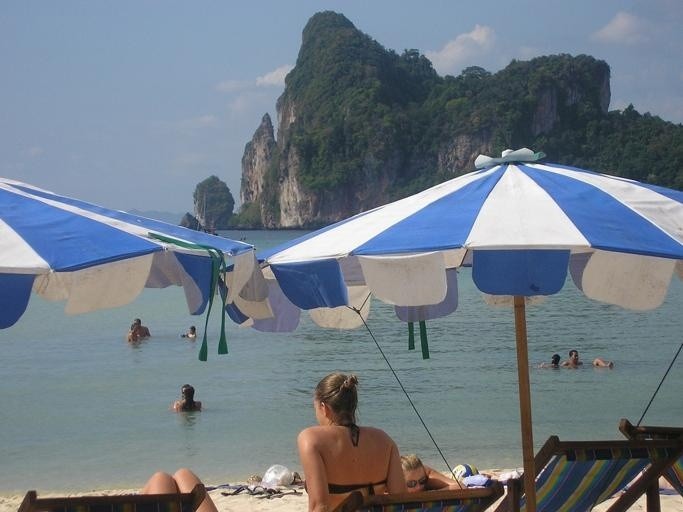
407 476 427 488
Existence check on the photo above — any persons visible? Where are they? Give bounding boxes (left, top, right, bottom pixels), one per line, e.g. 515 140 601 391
297 372 407 511
134 319 151 337
560 350 615 368
173 384 201 409
399 453 466 491
184 326 196 340
128 324 141 344
141 468 218 512
540 353 561 368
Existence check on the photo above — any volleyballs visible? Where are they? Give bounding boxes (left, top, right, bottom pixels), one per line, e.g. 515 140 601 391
451 464 479 482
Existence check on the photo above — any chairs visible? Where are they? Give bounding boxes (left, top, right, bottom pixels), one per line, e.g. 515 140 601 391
619 419 683 499
492 433 682 511
331 480 507 511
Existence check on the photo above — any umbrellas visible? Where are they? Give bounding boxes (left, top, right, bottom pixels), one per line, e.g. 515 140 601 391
0 175 274 330
218 147 683 512
